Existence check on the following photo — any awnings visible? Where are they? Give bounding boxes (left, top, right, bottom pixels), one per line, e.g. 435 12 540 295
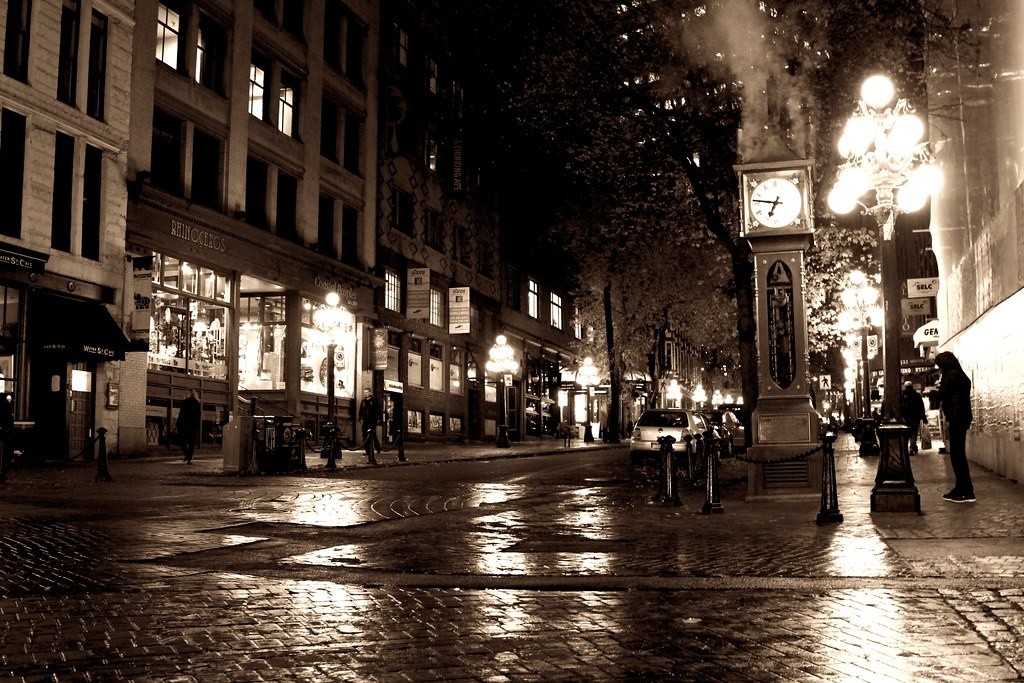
35 293 129 364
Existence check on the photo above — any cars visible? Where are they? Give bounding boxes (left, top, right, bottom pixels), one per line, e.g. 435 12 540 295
629 408 706 463
691 411 733 458
706 408 746 450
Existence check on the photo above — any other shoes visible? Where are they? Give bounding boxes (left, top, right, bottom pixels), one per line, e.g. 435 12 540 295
910 445 918 455
363 453 369 456
377 448 381 454
182 456 192 464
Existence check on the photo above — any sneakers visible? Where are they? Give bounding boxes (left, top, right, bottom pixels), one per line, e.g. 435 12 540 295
943 489 976 503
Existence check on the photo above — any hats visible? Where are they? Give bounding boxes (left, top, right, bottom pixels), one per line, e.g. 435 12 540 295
904 381 913 387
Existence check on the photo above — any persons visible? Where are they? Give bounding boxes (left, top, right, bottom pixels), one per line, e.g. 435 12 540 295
175 386 202 466
927 351 977 503
357 387 382 456
901 380 929 457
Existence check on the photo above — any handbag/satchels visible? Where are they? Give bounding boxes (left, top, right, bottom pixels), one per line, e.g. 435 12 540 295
921 423 931 450
